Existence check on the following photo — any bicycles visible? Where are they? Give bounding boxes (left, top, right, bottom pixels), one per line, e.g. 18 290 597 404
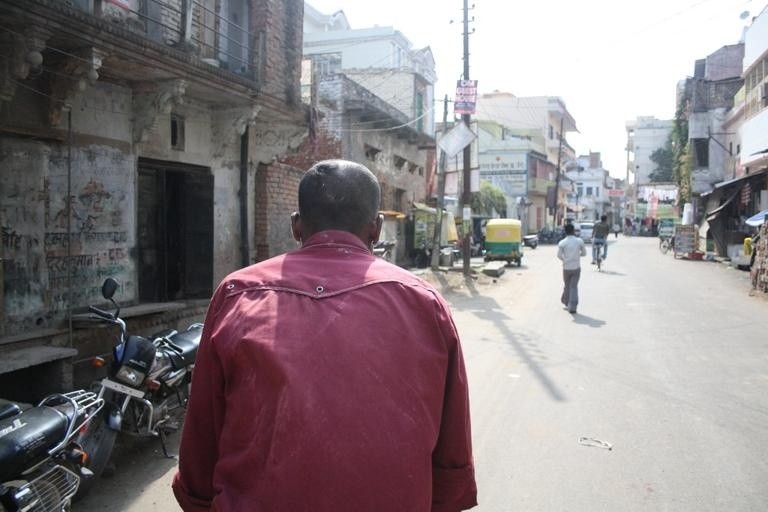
658 237 686 256
593 237 603 272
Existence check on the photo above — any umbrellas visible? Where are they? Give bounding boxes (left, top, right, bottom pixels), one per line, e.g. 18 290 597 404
744 208 768 227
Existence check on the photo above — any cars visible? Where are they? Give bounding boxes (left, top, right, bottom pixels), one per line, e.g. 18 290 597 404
579 223 595 243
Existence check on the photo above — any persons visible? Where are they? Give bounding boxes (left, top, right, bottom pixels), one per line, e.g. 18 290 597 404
591 215 608 265
612 222 620 238
557 225 588 313
747 224 762 279
172 160 479 511
